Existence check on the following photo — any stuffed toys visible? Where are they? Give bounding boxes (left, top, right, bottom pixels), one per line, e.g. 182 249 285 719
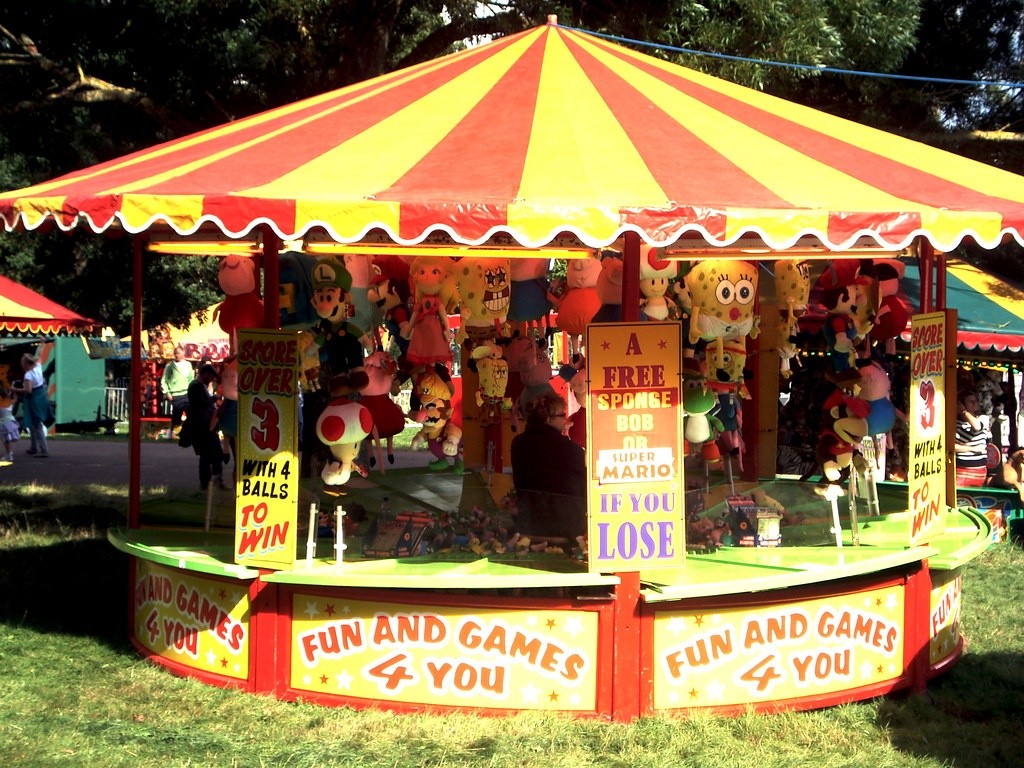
953 369 1024 430
214 242 911 549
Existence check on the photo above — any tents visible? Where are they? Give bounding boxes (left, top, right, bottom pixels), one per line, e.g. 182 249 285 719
787 253 1024 374
1 274 105 337
119 297 237 416
1 12 1024 548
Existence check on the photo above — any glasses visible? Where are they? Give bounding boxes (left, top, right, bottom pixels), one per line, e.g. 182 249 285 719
550 413 566 419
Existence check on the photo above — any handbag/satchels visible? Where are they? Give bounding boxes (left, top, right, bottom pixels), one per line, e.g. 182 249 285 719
179 419 193 447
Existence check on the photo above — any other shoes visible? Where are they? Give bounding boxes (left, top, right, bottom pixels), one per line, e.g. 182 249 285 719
213 479 232 492
167 429 173 439
25 448 48 458
4 453 14 461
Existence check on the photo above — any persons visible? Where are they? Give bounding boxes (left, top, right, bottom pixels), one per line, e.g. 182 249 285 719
180 364 230 492
510 391 587 542
10 336 57 457
0 378 21 461
954 388 990 470
160 346 195 441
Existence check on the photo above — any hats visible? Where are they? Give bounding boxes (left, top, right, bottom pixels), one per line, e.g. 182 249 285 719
205 364 218 376
